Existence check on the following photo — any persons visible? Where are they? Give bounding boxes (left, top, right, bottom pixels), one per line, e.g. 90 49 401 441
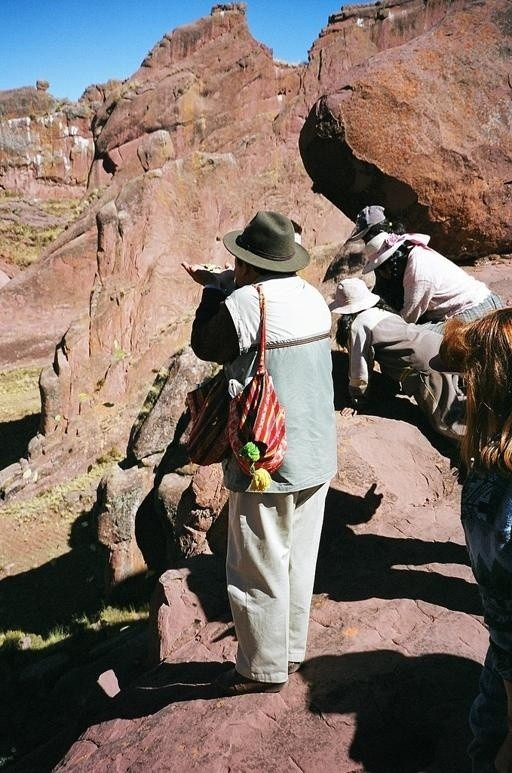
360 229 504 325
347 201 407 248
457 306 509 767
181 210 336 696
330 275 475 451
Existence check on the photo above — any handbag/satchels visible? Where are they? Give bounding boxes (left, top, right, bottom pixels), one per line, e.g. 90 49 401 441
184 366 247 467
227 373 286 480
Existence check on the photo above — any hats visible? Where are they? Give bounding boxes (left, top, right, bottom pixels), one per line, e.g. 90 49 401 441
347 205 388 238
357 232 407 274
330 276 382 314
222 209 311 274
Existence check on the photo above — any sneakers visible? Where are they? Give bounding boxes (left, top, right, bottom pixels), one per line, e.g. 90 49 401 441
213 668 285 694
288 661 301 674
